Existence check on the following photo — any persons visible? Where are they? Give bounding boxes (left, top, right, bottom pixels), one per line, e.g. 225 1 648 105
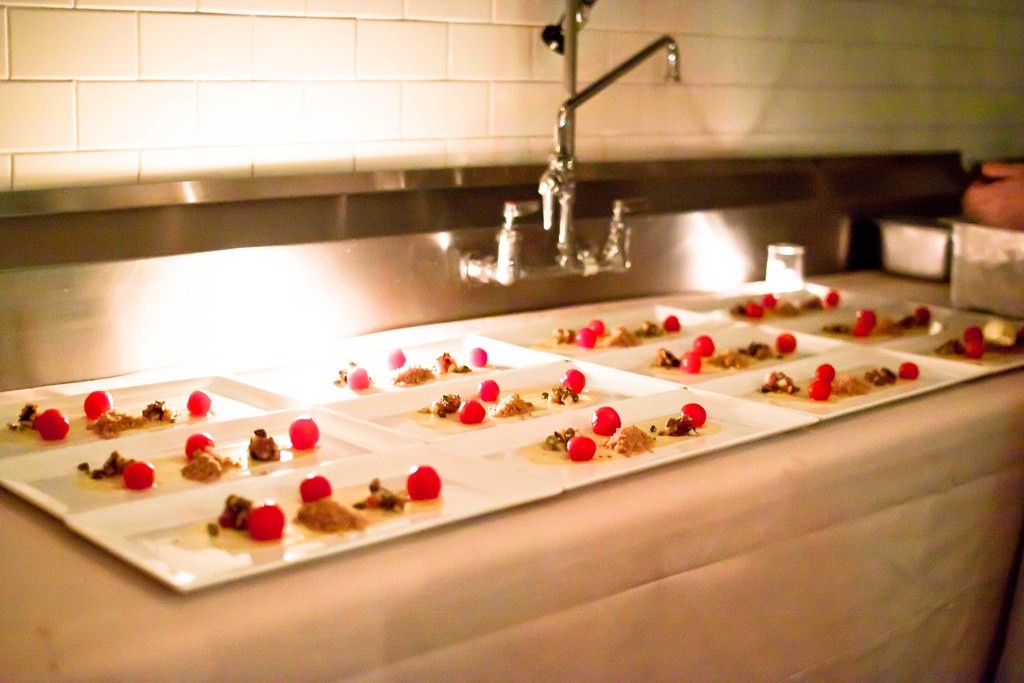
963 160 1024 230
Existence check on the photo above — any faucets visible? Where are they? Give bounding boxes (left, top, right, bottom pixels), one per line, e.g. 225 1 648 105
538 35 682 273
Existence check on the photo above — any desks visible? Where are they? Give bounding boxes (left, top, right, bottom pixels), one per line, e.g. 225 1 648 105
0 270 1024 683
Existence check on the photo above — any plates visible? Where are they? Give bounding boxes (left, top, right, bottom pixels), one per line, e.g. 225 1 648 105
576 326 848 387
448 386 820 494
697 348 988 421
64 443 564 595
764 300 977 346
319 355 687 445
699 284 863 325
490 304 748 357
881 314 1024 371
0 405 423 520
219 325 567 406
0 369 311 467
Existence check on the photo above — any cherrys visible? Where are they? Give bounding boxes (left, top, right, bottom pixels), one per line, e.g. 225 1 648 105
32 289 984 539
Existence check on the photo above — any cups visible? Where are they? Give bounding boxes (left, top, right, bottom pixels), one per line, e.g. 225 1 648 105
764 241 805 295
